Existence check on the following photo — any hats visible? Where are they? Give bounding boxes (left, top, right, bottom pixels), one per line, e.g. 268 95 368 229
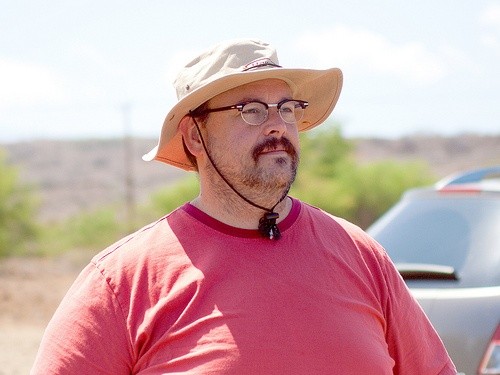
142 36 343 173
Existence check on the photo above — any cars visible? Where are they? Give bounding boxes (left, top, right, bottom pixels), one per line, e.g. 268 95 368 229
364 166 500 375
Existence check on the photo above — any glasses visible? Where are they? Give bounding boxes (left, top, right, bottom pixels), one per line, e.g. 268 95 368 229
192 98 309 126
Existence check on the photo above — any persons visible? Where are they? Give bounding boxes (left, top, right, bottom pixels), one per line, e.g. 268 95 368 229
29 40 462 375
